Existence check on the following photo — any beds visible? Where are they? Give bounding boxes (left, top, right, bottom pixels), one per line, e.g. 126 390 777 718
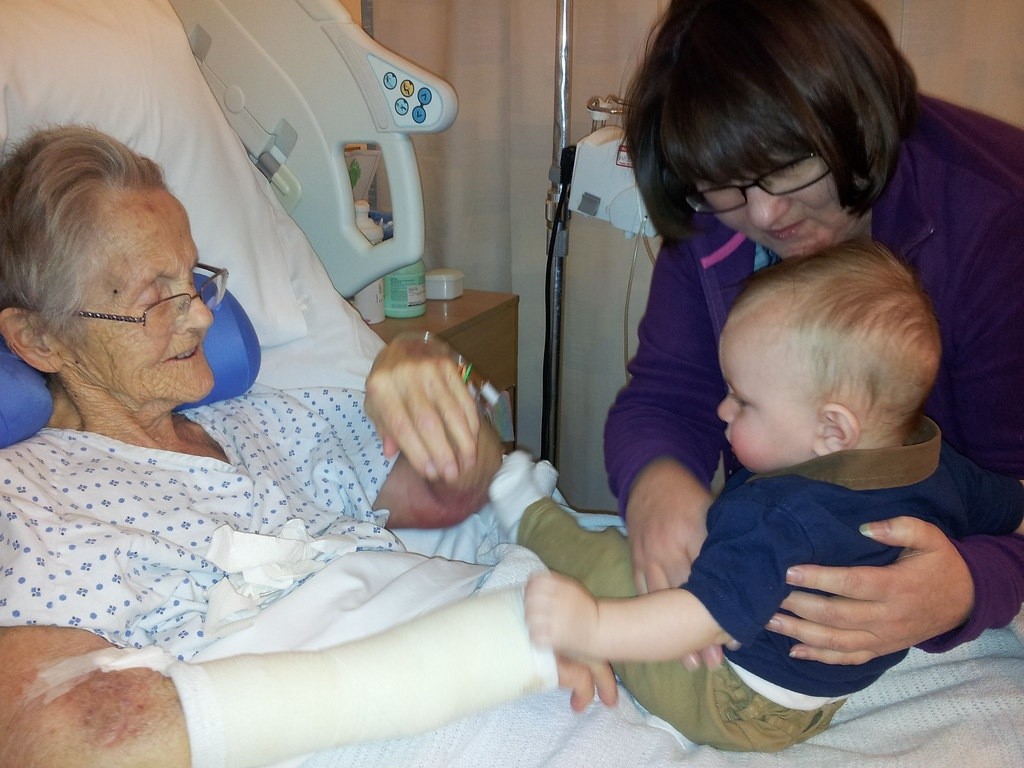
0 0 1024 768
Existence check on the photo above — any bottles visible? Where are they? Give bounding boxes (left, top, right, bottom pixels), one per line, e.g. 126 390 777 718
385 258 426 320
354 199 386 325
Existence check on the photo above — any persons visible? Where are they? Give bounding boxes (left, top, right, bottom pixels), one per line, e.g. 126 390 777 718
489 239 1024 754
0 127 1024 767
603 0 1024 664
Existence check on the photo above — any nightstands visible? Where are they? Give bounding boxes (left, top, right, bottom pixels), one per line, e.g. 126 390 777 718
358 292 521 454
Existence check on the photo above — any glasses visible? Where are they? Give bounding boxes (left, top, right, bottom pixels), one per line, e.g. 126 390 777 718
685 153 829 215
65 262 229 339
